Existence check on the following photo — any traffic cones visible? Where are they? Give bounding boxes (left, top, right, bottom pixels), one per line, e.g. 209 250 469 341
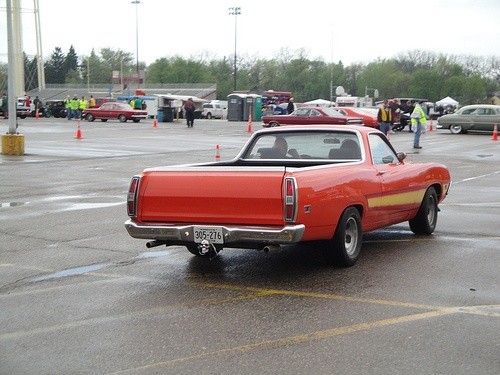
36 109 41 120
245 117 254 133
386 131 391 142
489 123 500 140
428 117 434 132
216 143 220 161
152 115 158 128
74 118 84 139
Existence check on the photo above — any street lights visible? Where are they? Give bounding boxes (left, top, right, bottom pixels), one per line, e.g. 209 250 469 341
228 5 242 91
131 0 141 73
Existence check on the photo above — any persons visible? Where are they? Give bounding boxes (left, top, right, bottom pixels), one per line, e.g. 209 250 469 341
184 98 195 127
410 103 426 149
378 100 392 137
1 92 41 119
287 98 295 115
63 94 95 120
128 97 146 110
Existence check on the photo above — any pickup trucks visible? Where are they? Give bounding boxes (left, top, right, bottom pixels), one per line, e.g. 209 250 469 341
123 114 452 269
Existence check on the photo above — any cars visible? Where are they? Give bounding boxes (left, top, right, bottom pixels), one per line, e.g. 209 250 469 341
261 107 364 127
0 96 228 120
436 105 500 134
329 106 380 129
260 89 442 122
82 101 147 123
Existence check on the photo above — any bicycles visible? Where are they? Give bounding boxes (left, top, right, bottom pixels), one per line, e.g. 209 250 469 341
392 114 411 131
